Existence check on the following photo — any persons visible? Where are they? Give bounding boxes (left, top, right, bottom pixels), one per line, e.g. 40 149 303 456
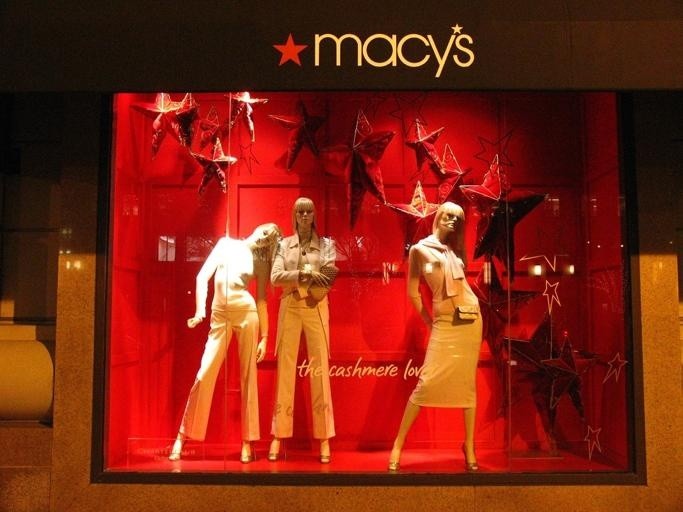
388 202 483 473
168 223 281 463
268 197 339 463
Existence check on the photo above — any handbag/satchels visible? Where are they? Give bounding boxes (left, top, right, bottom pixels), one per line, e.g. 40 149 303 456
309 265 340 302
457 306 478 320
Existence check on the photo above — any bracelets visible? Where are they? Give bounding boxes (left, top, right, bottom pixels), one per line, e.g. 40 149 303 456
305 270 312 278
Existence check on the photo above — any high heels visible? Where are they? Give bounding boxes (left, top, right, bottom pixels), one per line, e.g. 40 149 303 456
320 454 330 463
462 443 479 471
389 454 400 471
268 452 279 461
169 437 187 460
240 455 253 463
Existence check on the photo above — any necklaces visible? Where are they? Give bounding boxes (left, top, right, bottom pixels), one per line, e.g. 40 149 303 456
302 250 306 256
